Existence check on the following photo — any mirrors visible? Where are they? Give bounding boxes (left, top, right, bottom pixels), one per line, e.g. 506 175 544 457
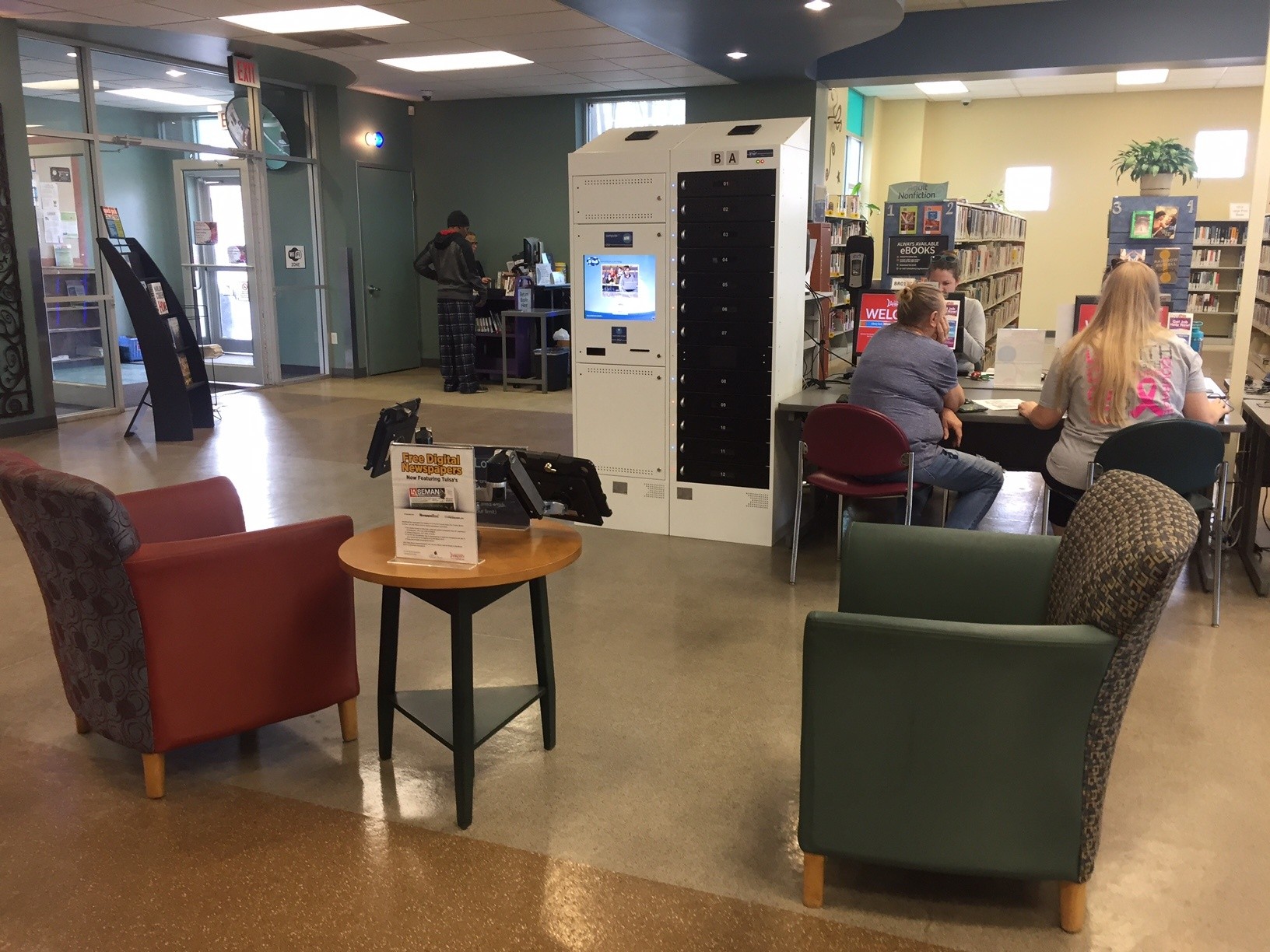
225 97 291 171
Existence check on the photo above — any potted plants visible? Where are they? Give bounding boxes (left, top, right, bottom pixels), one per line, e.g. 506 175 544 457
1113 137 1196 197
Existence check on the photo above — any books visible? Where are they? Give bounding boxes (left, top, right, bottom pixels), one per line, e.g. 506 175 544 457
1129 211 1155 238
827 192 859 213
898 205 918 234
1187 222 1247 345
1120 247 1181 284
957 205 1026 371
923 205 943 235
822 219 863 338
1152 205 1179 239
1167 312 1194 346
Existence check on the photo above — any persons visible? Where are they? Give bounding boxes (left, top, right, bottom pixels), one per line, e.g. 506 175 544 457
1019 259 1229 536
602 266 636 291
923 250 986 375
1152 210 1177 238
414 211 491 394
847 283 1004 530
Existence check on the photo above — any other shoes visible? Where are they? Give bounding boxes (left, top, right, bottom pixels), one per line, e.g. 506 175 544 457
460 384 488 394
898 492 924 524
443 379 461 392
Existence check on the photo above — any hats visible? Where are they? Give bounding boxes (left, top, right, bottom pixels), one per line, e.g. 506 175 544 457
447 211 470 227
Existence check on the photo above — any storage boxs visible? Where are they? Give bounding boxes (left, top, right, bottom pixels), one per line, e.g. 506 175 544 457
116 335 144 363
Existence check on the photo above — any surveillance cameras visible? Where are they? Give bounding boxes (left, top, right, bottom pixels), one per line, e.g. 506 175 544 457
962 98 971 106
421 91 433 101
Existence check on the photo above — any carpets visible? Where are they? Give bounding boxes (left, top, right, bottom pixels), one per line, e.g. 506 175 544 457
122 382 244 409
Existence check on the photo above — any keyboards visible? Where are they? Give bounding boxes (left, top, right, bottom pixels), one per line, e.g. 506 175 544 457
836 394 849 404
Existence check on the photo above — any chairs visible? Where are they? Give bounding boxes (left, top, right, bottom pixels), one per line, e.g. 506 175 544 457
0 446 362 798
788 403 953 585
1066 413 1227 629
796 466 1198 934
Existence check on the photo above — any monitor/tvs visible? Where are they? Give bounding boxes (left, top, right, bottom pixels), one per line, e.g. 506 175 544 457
852 289 964 352
1073 295 1173 337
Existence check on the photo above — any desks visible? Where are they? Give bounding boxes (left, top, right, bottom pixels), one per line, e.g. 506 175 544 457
778 373 1270 574
339 511 581 830
501 307 570 394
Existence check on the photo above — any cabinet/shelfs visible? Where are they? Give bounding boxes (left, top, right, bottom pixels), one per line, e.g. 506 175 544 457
884 199 1027 370
1107 196 1270 374
568 146 780 547
475 278 534 388
822 213 866 358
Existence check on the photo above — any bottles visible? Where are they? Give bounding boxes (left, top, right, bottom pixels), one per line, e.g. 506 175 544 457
555 262 567 283
